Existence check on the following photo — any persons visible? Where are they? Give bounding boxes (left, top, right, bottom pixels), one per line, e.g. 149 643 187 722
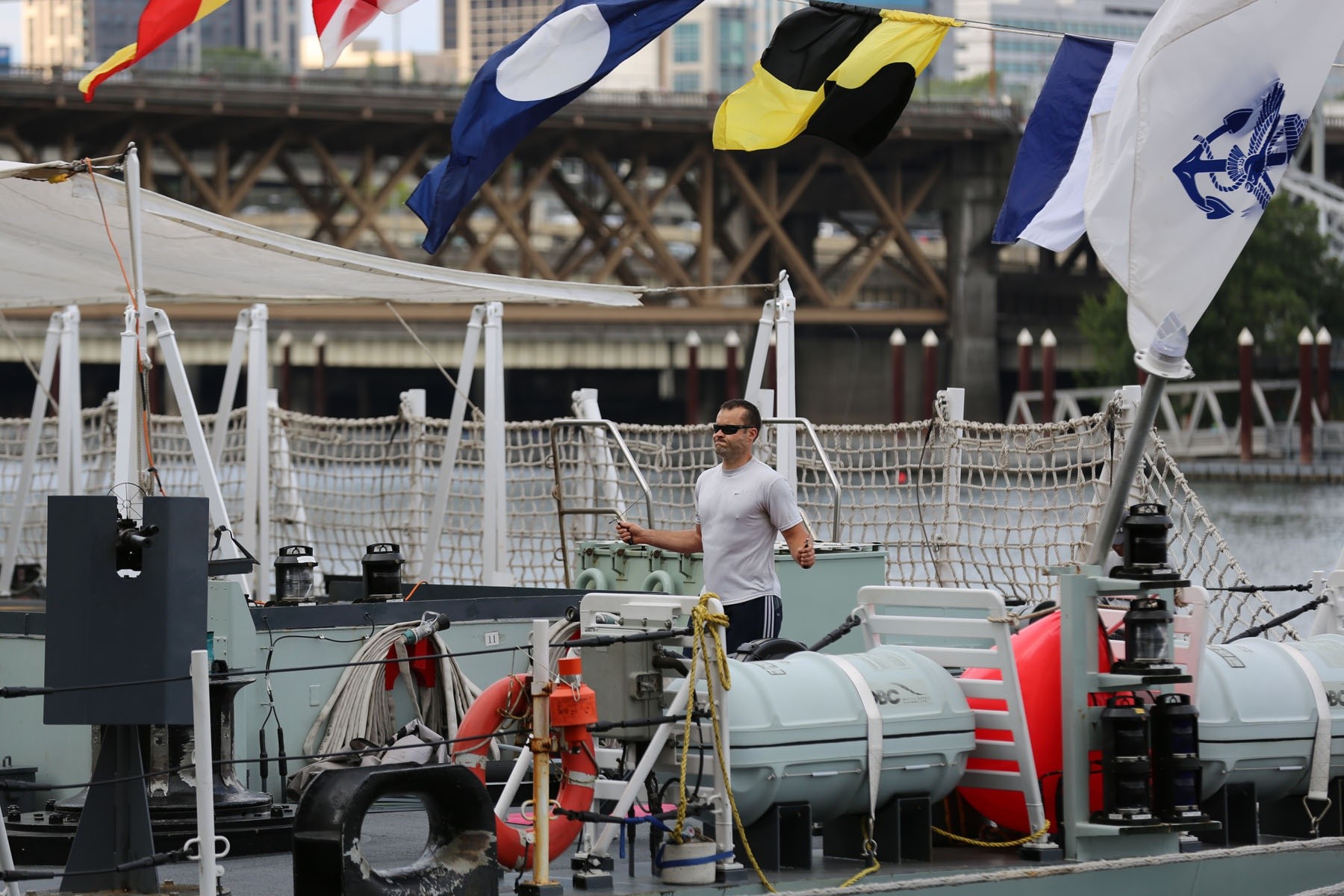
615 397 816 655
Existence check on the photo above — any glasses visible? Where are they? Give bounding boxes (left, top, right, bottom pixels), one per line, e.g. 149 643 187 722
713 424 759 435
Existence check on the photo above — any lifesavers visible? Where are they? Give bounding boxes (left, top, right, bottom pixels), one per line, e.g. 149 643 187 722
451 673 596 872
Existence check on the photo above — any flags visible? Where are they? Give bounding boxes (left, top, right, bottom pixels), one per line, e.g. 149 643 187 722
77 0 232 113
1078 1 1344 355
311 0 422 71
711 0 965 153
402 1 708 256
989 32 1136 255
